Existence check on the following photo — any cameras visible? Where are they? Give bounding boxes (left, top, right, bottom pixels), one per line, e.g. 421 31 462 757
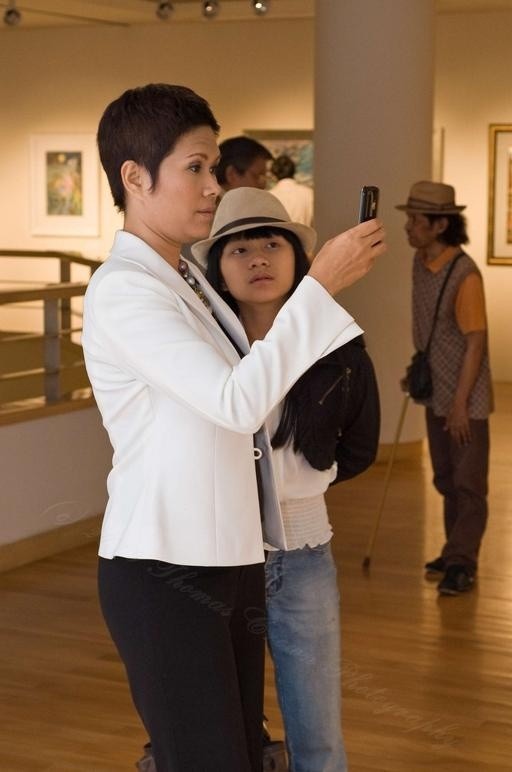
400 351 433 408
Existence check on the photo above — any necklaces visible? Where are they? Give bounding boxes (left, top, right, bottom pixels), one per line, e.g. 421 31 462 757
176 258 213 314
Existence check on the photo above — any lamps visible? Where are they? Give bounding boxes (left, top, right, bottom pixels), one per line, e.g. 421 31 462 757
150 2 275 30
4 0 23 29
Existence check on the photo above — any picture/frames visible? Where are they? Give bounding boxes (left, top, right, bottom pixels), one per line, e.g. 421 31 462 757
484 121 512 268
242 130 313 189
27 129 102 237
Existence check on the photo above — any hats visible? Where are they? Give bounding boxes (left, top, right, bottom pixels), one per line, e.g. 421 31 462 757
389 180 470 216
188 187 318 273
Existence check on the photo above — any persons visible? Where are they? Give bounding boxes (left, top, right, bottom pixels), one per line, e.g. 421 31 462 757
393 176 496 596
174 136 275 290
191 186 381 771
266 152 317 227
77 81 391 771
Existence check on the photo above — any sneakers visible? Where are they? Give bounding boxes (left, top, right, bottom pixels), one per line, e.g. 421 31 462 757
423 550 479 598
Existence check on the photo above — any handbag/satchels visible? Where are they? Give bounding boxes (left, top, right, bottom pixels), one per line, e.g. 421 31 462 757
135 732 290 772
404 352 433 401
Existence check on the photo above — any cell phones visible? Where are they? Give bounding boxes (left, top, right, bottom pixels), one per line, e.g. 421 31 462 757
358 184 379 226
